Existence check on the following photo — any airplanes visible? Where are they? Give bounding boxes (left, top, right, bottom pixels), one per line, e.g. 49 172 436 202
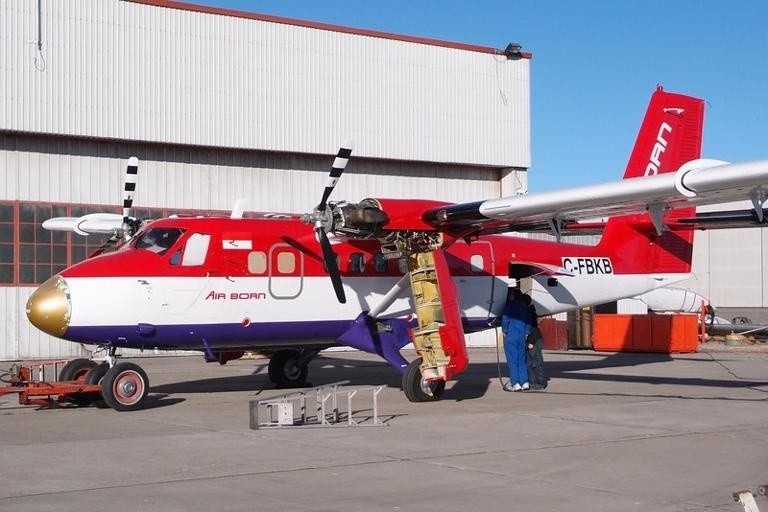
26 88 768 412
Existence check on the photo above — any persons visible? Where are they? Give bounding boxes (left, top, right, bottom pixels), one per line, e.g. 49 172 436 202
500 288 531 392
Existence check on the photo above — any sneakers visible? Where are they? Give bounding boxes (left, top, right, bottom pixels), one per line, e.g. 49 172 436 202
508 381 530 390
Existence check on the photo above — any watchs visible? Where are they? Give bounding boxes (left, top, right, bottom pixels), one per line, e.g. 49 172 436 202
521 295 549 390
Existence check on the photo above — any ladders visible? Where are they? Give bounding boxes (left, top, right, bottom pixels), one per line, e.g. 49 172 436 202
248 384 386 430
